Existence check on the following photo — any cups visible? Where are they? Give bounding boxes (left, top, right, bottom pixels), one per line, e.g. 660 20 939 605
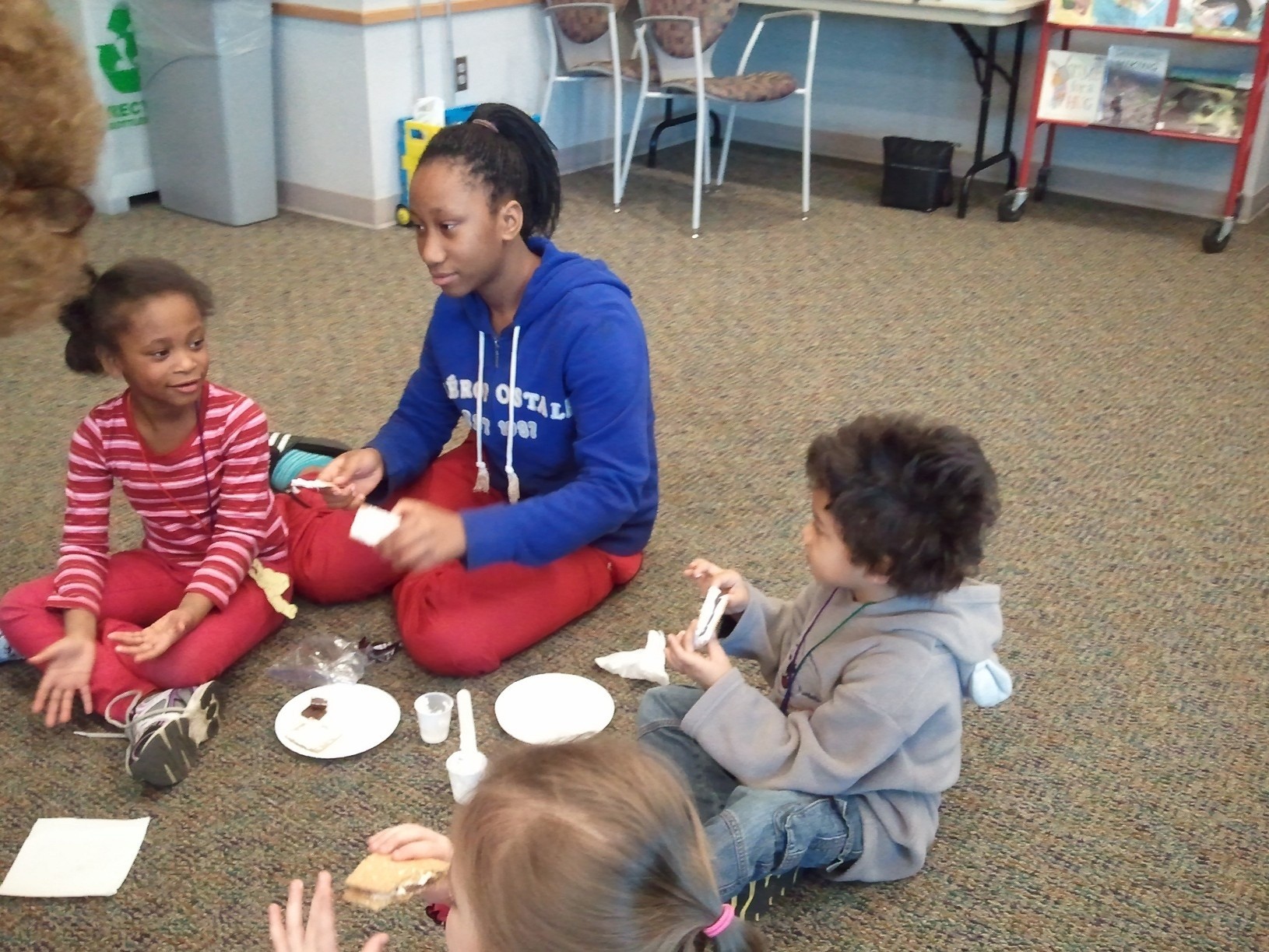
413 692 453 743
445 750 487 805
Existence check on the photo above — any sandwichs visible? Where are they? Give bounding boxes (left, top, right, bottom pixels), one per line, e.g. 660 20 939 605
694 587 729 649
342 852 450 915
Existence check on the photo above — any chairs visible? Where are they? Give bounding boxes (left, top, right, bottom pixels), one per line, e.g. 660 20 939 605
540 0 822 240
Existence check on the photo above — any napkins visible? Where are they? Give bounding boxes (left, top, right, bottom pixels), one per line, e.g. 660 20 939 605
0 816 151 898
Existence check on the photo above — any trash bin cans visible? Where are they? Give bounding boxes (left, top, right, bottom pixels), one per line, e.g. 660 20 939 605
127 0 278 227
47 0 159 215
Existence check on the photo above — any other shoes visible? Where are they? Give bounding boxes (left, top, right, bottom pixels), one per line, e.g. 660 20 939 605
725 865 801 922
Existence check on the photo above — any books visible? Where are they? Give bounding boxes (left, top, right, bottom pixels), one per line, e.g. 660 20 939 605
1037 0 1269 140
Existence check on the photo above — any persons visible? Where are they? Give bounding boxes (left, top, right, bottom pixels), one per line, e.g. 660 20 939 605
266 738 770 952
265 103 659 673
641 414 1013 903
0 258 297 789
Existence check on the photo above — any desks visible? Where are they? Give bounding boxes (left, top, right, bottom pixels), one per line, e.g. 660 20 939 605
647 0 1044 219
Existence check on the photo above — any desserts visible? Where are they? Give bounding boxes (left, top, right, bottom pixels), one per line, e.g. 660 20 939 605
289 698 339 752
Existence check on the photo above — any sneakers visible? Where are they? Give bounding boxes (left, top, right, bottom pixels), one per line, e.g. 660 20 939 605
72 679 226 786
0 633 27 662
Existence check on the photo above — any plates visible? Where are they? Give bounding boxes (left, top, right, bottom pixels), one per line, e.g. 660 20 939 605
275 683 401 759
494 673 614 744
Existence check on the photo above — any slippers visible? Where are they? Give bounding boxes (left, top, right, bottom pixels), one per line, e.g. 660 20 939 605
268 432 352 479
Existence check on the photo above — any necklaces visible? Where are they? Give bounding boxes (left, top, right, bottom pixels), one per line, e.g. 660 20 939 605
780 586 877 711
128 393 215 538
